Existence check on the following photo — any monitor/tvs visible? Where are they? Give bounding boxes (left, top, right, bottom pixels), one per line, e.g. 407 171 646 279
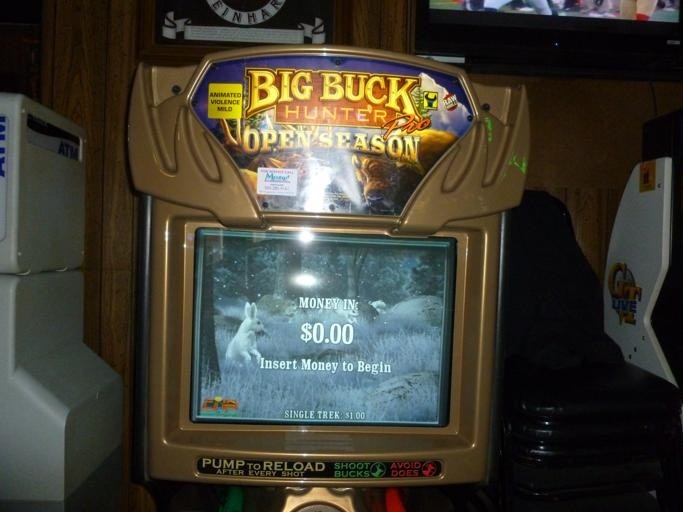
128 42 531 488
